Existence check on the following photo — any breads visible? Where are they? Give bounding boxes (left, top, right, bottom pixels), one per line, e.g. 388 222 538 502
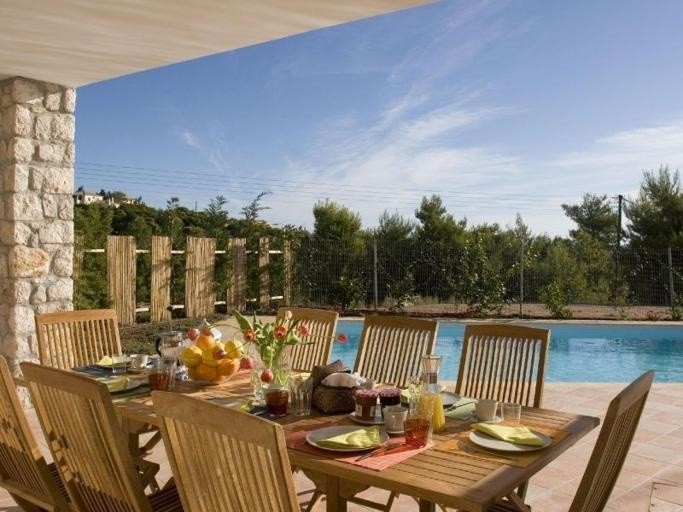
291 372 312 382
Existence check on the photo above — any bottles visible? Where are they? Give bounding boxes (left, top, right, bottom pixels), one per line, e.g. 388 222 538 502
402 375 422 422
419 354 447 433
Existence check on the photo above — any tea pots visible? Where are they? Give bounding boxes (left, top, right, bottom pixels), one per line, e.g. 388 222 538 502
154 328 185 366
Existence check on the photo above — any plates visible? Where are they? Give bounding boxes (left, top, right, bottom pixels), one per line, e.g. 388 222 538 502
371 422 404 434
440 391 460 407
101 355 153 369
468 426 552 453
306 424 390 453
93 378 142 393
125 366 153 373
468 413 504 424
347 408 385 427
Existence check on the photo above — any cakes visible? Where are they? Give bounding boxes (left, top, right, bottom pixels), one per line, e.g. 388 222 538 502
354 389 379 422
380 387 402 420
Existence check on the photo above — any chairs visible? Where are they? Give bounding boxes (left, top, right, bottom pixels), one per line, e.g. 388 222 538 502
18 360 184 512
451 369 655 512
306 313 440 512
412 323 550 504
0 353 159 512
270 307 340 477
150 390 302 512
34 308 162 493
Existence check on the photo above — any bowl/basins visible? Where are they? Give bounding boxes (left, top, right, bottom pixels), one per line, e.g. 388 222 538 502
178 354 243 386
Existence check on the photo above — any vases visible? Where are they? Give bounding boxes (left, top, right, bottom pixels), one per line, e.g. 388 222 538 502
259 367 288 418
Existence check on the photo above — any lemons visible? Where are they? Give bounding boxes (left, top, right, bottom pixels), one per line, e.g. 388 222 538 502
181 333 244 385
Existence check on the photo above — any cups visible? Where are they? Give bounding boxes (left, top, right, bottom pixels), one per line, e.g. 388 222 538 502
381 406 408 431
474 398 498 420
147 357 170 397
500 404 523 425
262 383 289 418
287 378 316 418
407 394 434 448
402 410 434 448
158 357 178 393
111 353 125 375
129 353 149 369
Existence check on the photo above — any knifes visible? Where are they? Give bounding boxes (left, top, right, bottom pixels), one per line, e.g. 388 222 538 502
354 441 407 463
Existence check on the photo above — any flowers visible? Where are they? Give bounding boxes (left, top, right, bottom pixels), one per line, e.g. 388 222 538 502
205 307 347 388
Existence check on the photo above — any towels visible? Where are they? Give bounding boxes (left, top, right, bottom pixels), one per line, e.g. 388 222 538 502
316 425 383 449
234 399 256 413
104 378 130 392
96 355 133 368
399 384 446 403
471 422 544 446
296 371 312 383
